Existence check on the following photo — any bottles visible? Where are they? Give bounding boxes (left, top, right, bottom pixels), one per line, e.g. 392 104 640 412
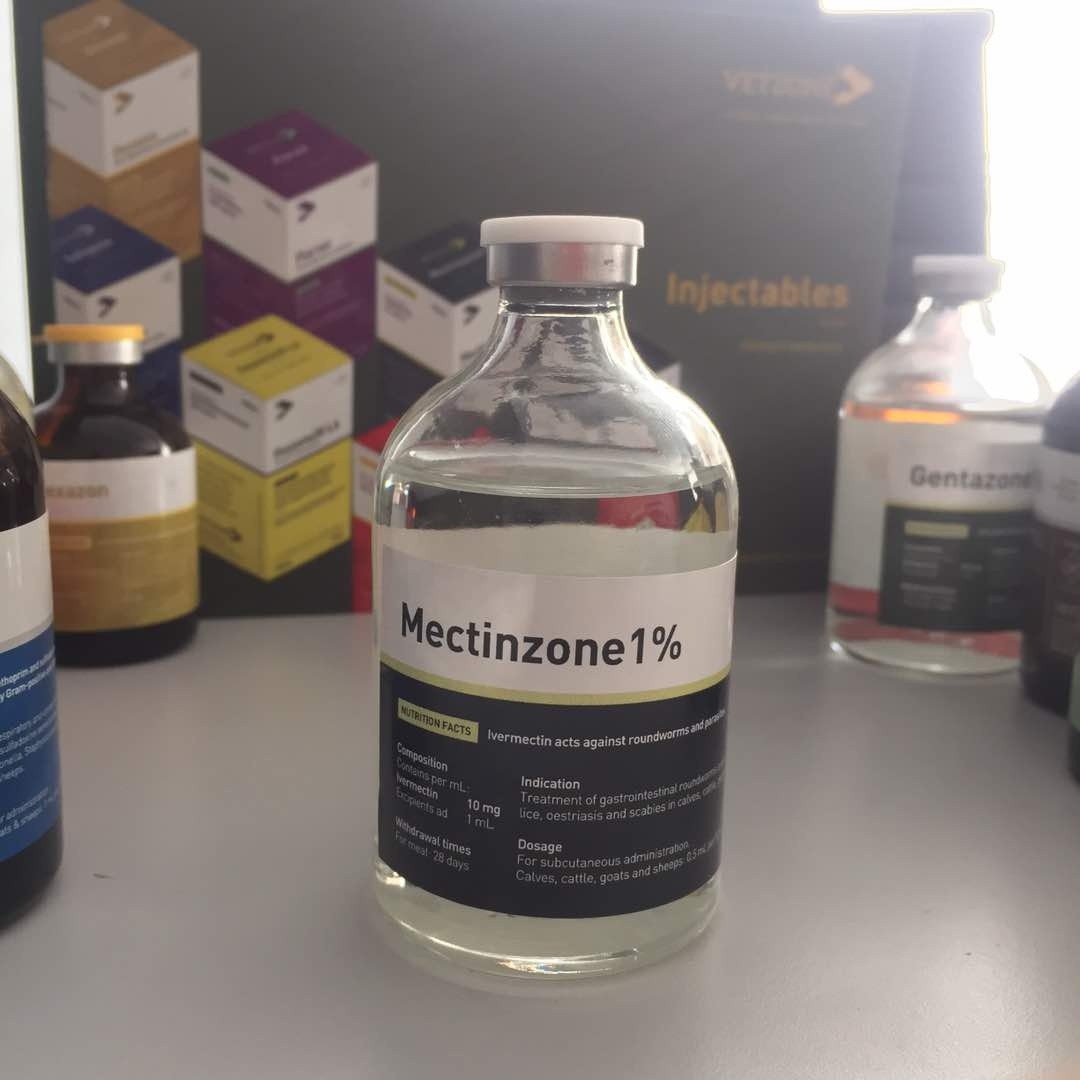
823 254 1055 684
32 320 202 668
0 389 65 938
368 214 740 983
1019 375 1079 710
